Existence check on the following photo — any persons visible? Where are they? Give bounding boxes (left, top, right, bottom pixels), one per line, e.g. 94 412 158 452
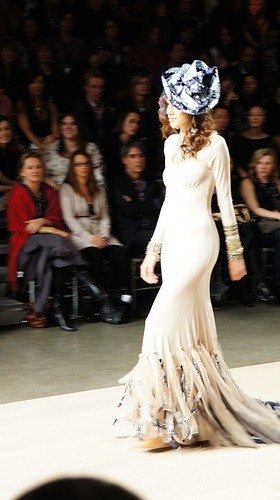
15 476 142 500
0 0 280 331
111 59 280 452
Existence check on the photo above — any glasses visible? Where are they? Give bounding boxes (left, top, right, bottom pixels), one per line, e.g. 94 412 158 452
246 112 265 119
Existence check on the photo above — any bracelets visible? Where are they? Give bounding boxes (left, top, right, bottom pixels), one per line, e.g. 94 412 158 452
227 254 244 260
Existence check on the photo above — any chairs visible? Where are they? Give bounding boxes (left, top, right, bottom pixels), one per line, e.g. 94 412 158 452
15 246 280 317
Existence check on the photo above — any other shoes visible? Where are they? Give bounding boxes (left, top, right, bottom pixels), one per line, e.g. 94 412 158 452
135 428 196 452
254 281 275 303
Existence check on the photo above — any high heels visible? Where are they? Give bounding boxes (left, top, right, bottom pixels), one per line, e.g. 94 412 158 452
80 278 111 302
50 299 75 331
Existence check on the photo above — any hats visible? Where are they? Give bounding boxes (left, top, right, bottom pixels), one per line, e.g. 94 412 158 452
160 59 221 116
